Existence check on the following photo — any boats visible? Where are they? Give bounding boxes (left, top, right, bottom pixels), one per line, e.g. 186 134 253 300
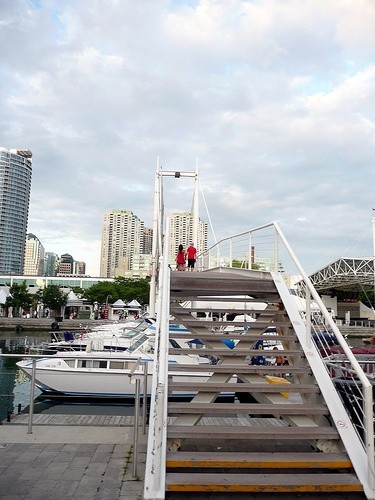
222 314 285 363
15 295 239 400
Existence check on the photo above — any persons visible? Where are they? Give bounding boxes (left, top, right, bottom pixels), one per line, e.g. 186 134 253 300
273 346 287 379
21 307 140 321
176 262 186 272
185 242 197 272
175 243 186 272
